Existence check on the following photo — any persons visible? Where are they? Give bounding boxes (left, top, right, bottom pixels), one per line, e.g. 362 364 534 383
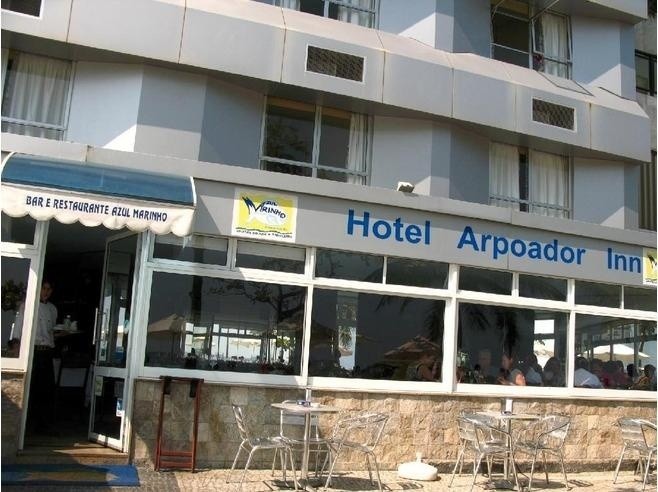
6 276 68 408
387 344 657 390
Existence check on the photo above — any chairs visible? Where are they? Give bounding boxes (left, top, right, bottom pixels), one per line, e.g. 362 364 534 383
615 416 657 491
231 403 389 491
448 412 571 491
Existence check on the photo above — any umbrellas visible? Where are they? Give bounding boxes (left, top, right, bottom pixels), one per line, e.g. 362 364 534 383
149 312 207 362
255 313 348 370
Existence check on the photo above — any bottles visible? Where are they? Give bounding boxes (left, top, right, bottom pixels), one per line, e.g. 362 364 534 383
63 314 71 331
